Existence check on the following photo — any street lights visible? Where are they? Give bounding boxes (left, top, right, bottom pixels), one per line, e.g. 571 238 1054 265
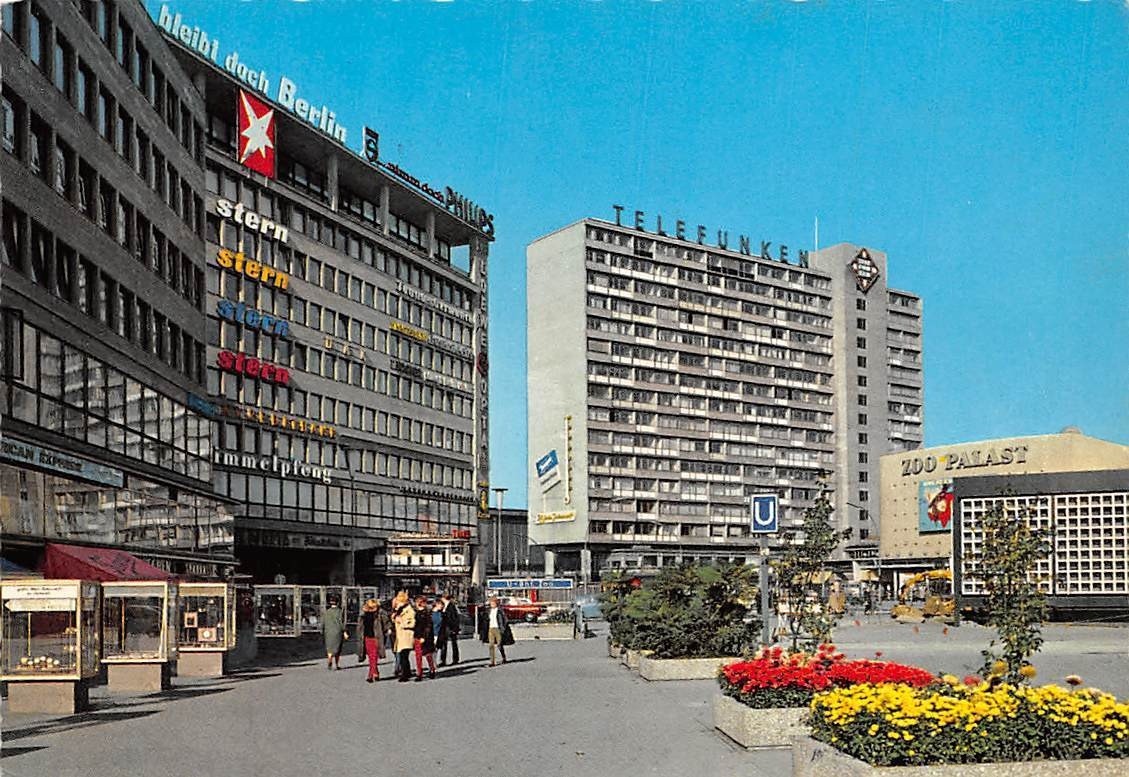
492 488 509 575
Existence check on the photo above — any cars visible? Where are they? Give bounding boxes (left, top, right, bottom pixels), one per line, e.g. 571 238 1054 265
468 597 543 623
564 592 605 618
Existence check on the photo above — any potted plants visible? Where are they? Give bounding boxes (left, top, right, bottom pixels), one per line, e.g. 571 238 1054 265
605 565 759 680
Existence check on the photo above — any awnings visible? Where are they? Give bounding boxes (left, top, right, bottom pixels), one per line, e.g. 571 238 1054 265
43 544 169 583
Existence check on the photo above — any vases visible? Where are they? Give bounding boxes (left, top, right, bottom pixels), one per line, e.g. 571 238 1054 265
712 687 812 752
793 737 1129 777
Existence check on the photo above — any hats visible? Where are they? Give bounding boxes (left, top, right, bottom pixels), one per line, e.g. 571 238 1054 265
394 591 410 605
362 598 379 612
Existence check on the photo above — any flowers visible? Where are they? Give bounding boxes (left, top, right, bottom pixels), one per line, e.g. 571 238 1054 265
718 646 1129 766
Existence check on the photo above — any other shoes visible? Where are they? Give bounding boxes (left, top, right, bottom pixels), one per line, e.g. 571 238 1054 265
415 677 421 680
430 669 435 679
438 662 446 666
328 662 332 667
399 675 409 681
375 676 379 681
367 678 373 682
502 659 506 663
489 664 496 666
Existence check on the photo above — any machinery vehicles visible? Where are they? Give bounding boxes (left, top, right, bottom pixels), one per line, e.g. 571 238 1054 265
892 569 954 621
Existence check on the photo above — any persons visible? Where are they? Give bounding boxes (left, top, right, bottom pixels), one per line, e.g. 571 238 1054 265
755 590 778 617
865 588 871 614
321 593 514 682
